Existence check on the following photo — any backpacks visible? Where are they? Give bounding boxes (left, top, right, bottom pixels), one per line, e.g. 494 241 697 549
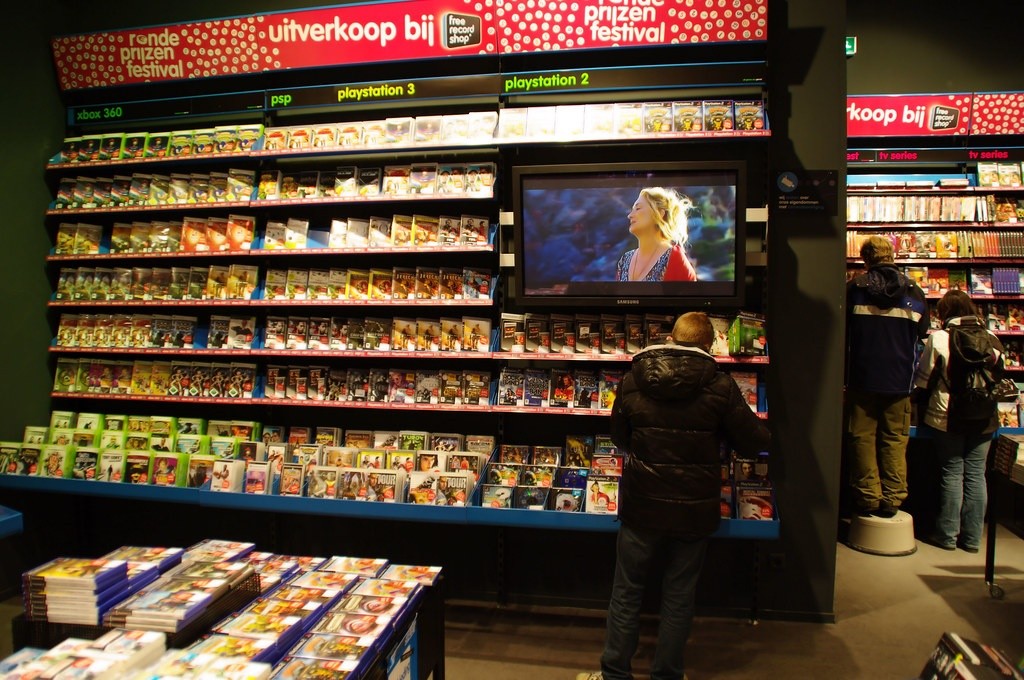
943 320 1019 403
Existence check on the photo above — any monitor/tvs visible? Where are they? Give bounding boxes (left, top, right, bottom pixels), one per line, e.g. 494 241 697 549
512 160 749 309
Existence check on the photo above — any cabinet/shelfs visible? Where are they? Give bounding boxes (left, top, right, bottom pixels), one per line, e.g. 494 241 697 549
846 148 1023 438
0 61 782 540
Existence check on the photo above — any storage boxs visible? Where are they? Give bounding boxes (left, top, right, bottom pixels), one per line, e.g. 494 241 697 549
729 319 766 356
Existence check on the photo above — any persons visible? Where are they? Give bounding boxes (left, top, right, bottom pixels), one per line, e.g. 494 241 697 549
743 461 757 484
911 290 1005 554
440 217 488 245
0 259 622 521
575 311 773 680
845 234 930 517
616 186 702 280
436 164 492 193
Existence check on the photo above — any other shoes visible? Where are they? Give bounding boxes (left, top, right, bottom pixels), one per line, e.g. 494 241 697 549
848 500 898 515
957 538 978 551
575 671 603 680
929 533 956 549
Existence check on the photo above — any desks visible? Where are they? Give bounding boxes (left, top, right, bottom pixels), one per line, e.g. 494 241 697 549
13 574 445 680
985 466 1024 600
